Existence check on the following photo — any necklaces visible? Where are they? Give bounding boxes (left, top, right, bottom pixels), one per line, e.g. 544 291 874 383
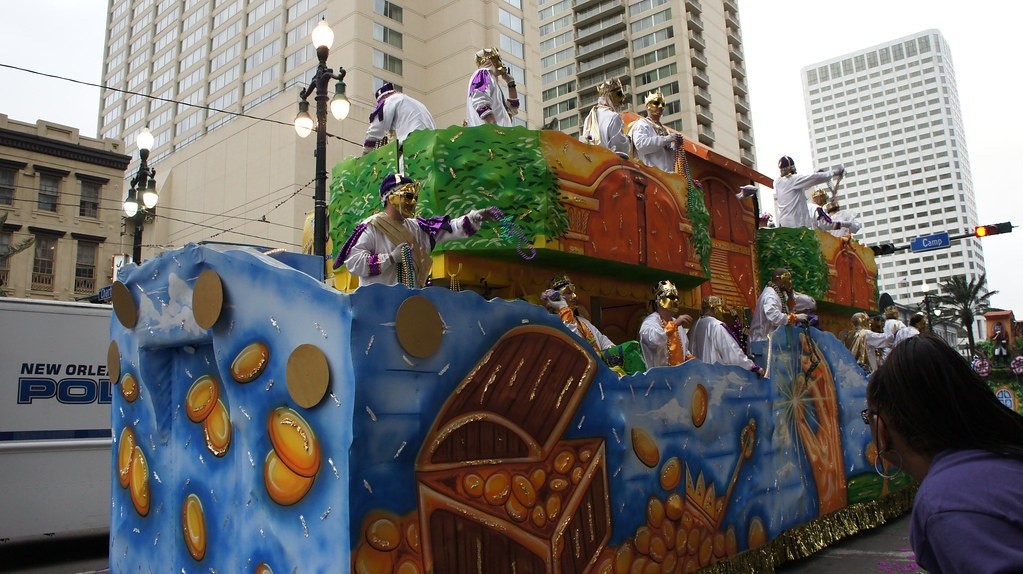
396 245 415 291
823 175 842 202
766 280 786 312
376 137 388 148
648 116 694 206
488 205 537 260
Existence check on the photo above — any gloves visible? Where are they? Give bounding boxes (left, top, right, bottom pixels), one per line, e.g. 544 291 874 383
795 313 808 322
543 289 568 311
832 166 845 176
469 208 497 223
391 242 414 264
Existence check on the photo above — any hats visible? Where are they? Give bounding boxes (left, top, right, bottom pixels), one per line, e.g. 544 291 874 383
375 82 396 102
778 156 795 170
378 173 414 208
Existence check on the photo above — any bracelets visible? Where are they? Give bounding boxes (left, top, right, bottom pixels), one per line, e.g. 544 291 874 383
506 81 516 88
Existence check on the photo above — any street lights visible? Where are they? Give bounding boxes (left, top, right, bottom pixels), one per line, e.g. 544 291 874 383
292 16 351 286
916 293 941 333
120 120 158 268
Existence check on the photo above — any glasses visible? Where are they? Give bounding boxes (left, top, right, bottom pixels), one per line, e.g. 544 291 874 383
664 294 680 302
393 191 419 202
860 408 894 440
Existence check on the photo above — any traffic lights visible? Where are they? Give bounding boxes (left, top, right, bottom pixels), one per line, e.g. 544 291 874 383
868 243 895 256
975 221 1013 238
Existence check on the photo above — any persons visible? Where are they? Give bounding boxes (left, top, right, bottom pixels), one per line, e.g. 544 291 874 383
990 322 1010 369
628 88 683 174
806 188 862 237
463 46 520 127
861 332 1023 574
582 77 632 159
747 267 816 356
638 278 696 371
548 273 626 381
331 175 498 288
687 295 765 376
773 155 843 229
362 82 436 178
846 292 926 377
